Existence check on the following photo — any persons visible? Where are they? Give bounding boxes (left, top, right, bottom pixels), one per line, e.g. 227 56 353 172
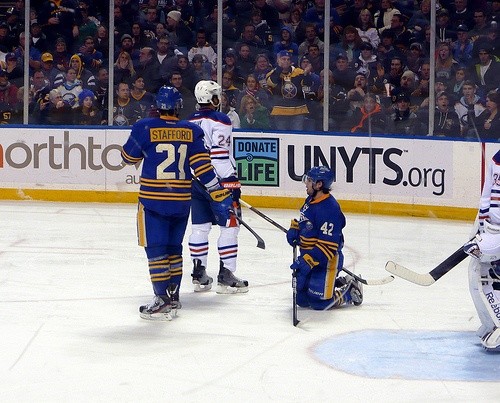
0 0 500 142
120 86 234 313
286 166 361 310
464 148 500 348
188 80 250 287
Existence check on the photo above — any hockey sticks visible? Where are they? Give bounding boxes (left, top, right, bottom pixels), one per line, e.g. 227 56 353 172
190 173 265 250
291 239 314 331
238 199 395 285
385 237 477 286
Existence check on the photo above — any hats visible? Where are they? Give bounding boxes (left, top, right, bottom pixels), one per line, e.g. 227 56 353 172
79 90 94 106
280 50 292 57
0 20 9 29
436 91 449 101
6 53 17 61
226 47 236 56
299 55 312 66
3 7 18 16
401 70 415 83
69 53 86 79
121 34 132 43
41 53 53 62
456 24 468 33
167 11 181 23
336 53 348 60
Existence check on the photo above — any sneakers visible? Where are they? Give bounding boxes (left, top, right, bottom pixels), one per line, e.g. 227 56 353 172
344 273 361 285
345 279 363 306
139 286 172 321
168 283 182 317
190 259 213 291
216 261 249 294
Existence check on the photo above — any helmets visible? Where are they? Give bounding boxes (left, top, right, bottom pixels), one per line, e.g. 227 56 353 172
156 86 183 111
195 80 222 105
307 166 333 189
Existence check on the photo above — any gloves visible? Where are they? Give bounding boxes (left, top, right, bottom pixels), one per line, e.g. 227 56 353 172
222 176 242 205
286 220 300 247
208 186 234 219
291 251 320 277
